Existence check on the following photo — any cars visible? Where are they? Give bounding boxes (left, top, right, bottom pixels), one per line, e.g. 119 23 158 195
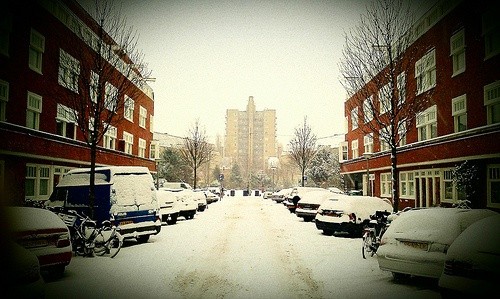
156 182 219 226
377 207 500 283
272 186 349 222
0 236 47 299
0 205 73 279
314 196 394 239
438 215 500 299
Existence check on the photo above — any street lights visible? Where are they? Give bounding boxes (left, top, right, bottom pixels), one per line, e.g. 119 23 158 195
155 158 162 191
362 153 374 196
270 166 277 193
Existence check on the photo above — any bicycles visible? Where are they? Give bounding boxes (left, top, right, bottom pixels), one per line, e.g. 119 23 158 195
23 198 123 259
361 199 472 259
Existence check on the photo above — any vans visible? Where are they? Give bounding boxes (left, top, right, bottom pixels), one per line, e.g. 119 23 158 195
48 165 162 248
